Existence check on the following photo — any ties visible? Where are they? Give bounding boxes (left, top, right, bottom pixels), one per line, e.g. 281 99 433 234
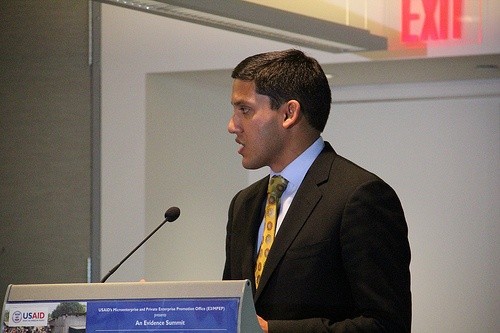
252 175 287 288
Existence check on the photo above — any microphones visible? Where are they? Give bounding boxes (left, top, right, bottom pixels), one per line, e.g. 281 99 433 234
100 207 180 286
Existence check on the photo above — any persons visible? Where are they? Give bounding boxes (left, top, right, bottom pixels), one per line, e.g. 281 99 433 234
219 48 414 333
